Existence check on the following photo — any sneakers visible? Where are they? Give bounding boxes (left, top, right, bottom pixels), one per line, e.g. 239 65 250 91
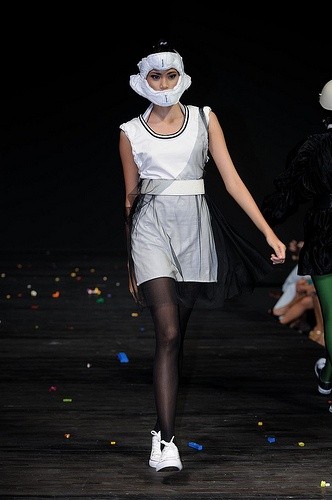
148 430 183 472
315 358 331 394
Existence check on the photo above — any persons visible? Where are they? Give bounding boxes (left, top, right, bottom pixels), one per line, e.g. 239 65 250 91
264 79 332 414
119 38 287 472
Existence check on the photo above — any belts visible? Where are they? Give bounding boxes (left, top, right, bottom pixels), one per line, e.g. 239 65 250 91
141 179 206 195
